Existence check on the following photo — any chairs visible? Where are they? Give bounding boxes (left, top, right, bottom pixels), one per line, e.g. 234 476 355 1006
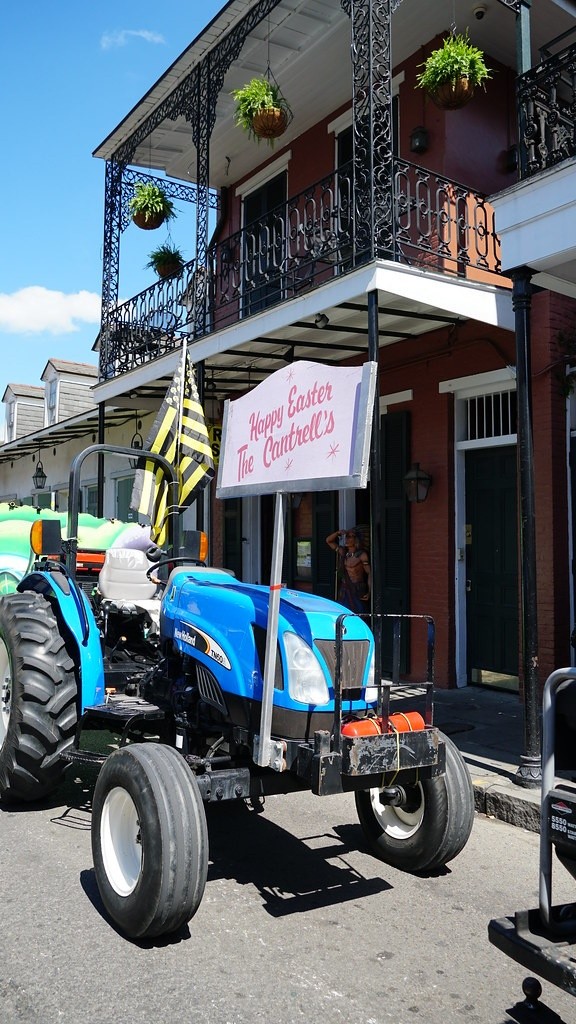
98 549 158 612
242 224 409 314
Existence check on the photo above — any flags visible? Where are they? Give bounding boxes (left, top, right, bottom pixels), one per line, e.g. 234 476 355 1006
129 348 215 546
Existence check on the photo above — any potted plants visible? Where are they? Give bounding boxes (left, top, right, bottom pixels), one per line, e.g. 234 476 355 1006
411 24 498 111
141 241 188 282
228 73 289 150
129 183 183 232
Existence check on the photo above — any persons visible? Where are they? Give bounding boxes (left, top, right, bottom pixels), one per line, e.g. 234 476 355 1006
326 526 371 613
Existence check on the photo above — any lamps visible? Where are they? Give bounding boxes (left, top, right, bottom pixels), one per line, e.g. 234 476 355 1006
402 462 431 503
282 347 296 364
205 378 216 391
129 389 137 400
128 410 144 469
313 313 330 328
409 125 429 153
507 144 519 173
31 446 48 490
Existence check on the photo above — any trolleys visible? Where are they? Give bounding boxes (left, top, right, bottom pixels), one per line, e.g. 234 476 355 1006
486 664 576 1024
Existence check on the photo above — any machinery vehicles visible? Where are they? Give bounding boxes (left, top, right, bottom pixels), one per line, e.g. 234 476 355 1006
0 442 476 944
